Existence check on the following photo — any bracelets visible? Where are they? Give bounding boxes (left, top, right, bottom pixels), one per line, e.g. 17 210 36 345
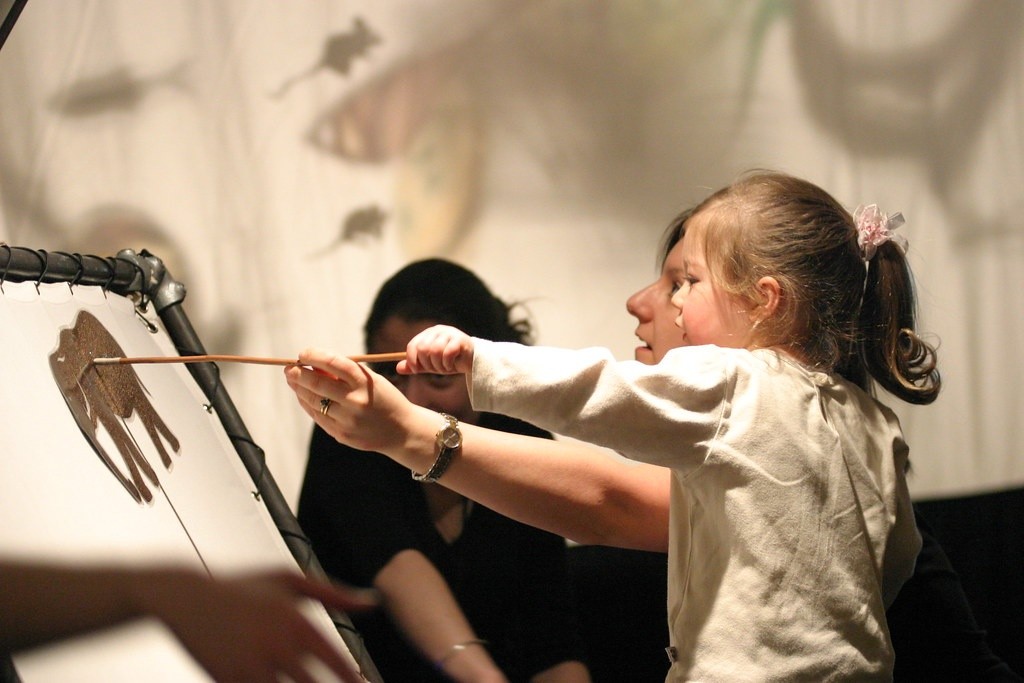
433 639 490 669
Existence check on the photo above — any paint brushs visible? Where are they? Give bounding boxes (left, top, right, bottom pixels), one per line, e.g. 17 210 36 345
94 350 405 367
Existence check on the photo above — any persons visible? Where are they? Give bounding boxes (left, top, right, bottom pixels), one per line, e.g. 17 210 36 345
397 172 940 683
293 259 643 683
0 550 382 683
284 207 691 552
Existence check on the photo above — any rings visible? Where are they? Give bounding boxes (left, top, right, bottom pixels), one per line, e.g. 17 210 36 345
319 397 331 414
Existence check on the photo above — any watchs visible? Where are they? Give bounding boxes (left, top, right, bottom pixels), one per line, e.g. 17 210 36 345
411 412 463 485
